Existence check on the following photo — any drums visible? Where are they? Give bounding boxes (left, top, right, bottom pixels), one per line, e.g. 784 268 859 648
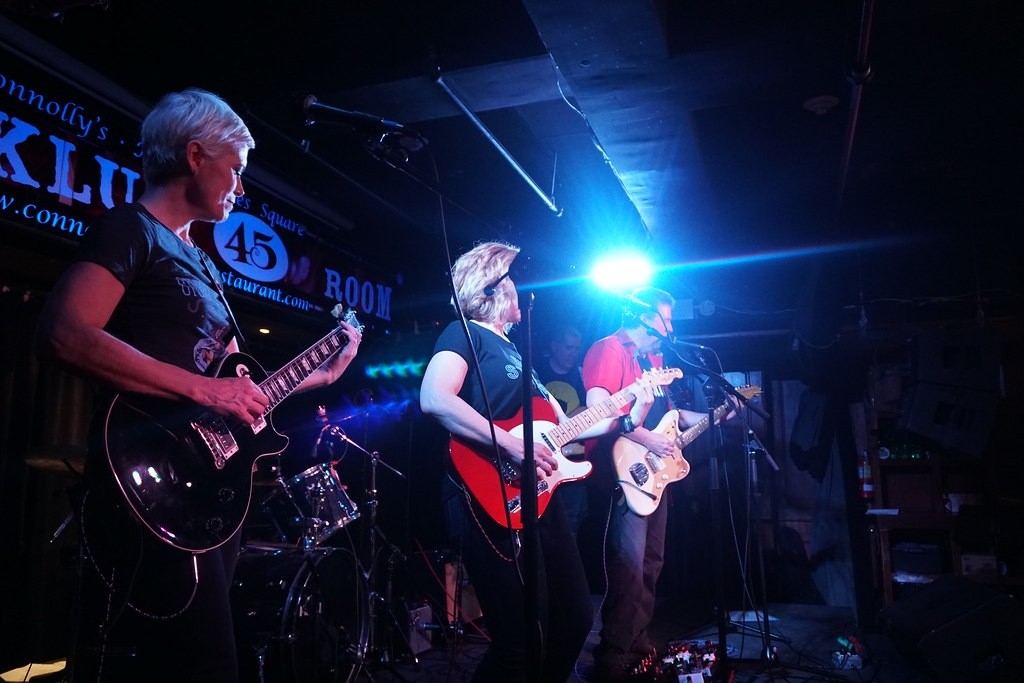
230 541 371 683
258 462 361 546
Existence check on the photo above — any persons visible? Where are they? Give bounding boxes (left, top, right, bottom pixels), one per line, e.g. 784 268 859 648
419 244 656 683
54 93 364 683
581 284 743 683
535 323 589 417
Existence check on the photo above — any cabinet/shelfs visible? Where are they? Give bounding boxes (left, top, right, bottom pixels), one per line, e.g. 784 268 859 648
844 315 1024 610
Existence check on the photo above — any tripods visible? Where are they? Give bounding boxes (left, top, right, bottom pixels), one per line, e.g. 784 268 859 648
666 381 850 683
367 514 421 668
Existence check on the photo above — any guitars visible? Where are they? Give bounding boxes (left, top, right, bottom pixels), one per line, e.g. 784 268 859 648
448 366 684 531
86 303 366 552
611 384 763 517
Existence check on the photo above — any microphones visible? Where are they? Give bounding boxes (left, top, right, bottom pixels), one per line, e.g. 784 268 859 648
600 278 658 315
299 93 400 132
667 334 709 351
416 622 456 633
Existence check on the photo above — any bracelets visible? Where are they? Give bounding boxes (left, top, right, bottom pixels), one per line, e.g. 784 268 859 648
622 415 632 433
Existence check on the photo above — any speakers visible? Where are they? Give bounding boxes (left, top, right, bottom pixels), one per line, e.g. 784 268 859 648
892 384 1002 463
874 573 1024 683
860 599 892 634
406 601 432 654
892 322 1006 390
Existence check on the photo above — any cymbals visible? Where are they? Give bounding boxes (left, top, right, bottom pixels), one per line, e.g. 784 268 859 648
276 409 366 437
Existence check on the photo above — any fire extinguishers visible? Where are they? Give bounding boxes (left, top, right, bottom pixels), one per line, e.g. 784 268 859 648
854 451 876 502
